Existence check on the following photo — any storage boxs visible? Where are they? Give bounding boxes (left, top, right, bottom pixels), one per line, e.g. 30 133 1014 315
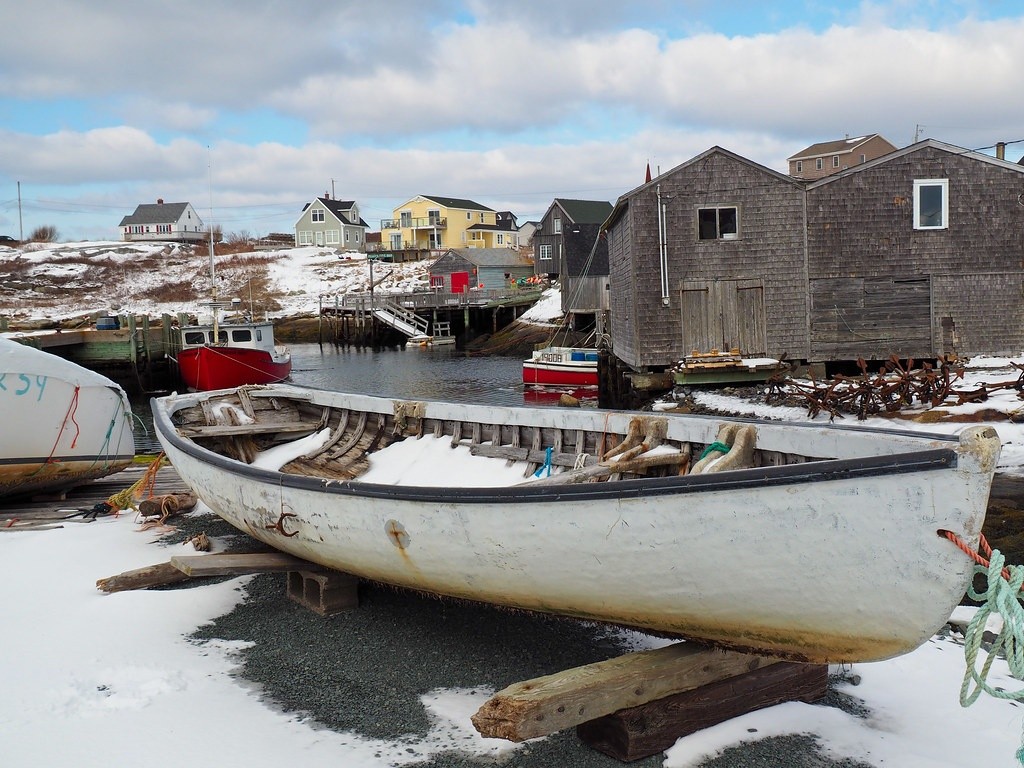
585 353 598 361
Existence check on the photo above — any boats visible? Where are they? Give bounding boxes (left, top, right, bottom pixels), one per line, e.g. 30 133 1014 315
0 330 137 504
177 146 293 399
523 356 598 390
149 378 1001 666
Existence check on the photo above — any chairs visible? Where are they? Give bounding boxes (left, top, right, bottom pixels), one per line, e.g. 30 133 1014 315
384 221 399 228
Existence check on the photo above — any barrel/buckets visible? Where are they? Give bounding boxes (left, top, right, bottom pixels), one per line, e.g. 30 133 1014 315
586 353 599 361
571 352 585 361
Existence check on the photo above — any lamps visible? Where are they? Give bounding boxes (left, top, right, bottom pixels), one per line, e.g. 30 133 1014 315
572 227 580 233
536 224 544 232
